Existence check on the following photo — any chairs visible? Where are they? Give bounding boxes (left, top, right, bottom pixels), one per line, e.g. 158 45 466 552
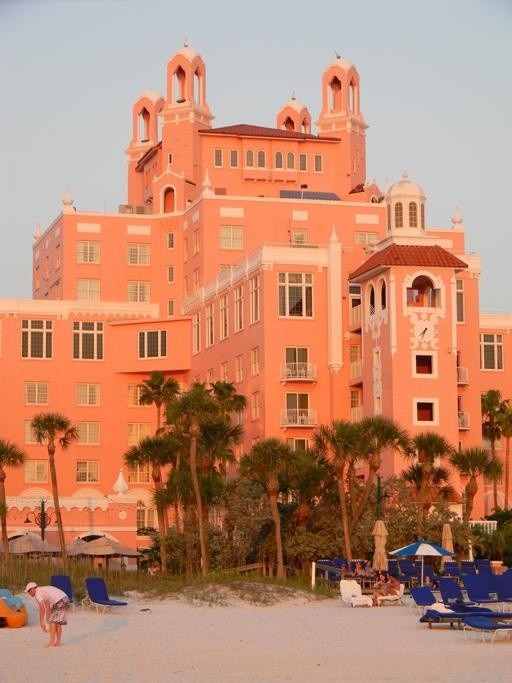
316 557 512 644
52 575 128 614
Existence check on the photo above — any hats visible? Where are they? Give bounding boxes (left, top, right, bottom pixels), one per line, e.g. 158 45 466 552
24 582 37 592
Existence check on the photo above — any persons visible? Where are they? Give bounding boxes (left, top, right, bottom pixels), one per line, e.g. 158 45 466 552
148 561 162 578
336 558 401 608
25 580 69 648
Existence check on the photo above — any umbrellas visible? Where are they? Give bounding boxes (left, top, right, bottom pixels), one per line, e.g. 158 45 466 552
388 539 458 588
